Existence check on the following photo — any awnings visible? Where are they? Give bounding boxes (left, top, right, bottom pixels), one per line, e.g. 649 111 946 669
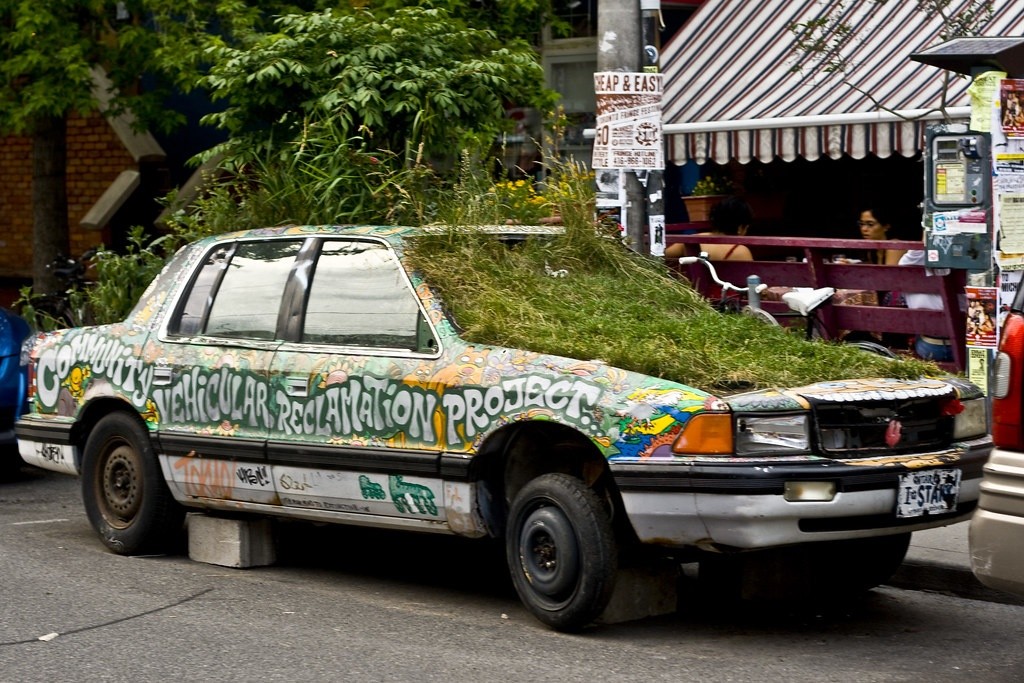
583 0 1024 167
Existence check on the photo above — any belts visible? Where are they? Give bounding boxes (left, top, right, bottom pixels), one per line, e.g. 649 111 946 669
919 335 952 346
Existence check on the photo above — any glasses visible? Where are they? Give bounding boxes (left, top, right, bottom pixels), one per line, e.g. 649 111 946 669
859 221 878 228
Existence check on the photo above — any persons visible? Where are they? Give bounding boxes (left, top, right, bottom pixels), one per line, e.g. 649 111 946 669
836 208 911 352
1003 92 1024 127
664 196 797 301
967 300 994 335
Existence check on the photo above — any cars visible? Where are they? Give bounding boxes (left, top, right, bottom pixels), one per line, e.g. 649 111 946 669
14 226 996 633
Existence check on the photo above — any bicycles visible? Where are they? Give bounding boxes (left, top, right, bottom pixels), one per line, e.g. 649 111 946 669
680 251 900 363
26 246 97 331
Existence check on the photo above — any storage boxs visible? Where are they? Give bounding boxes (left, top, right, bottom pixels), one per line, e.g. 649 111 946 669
187 514 282 568
